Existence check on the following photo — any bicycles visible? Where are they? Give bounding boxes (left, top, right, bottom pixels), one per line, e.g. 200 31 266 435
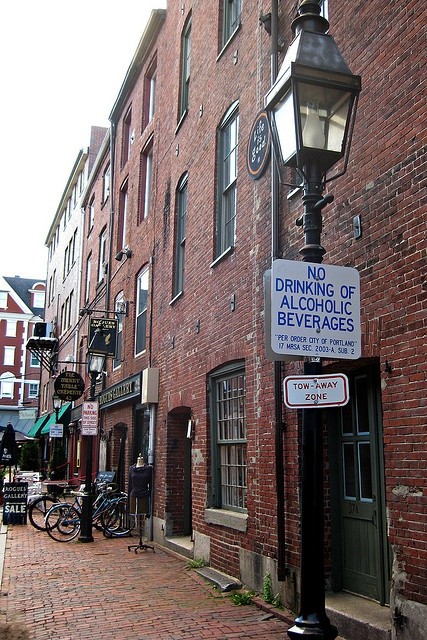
27 479 136 541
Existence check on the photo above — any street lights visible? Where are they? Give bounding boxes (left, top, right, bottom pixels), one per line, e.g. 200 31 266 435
52 391 62 512
79 350 107 543
262 3 362 638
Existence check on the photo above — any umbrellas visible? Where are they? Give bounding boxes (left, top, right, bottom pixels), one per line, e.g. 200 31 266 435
0 420 18 483
